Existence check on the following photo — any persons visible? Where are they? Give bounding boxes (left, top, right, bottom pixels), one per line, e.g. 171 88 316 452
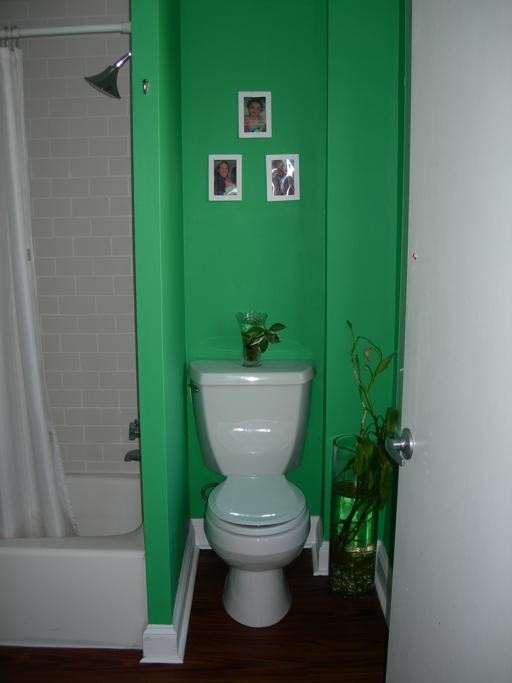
215 161 237 195
272 160 295 195
245 100 266 132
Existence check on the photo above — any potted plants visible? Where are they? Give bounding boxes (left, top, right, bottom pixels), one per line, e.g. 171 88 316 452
236 310 287 368
329 315 405 597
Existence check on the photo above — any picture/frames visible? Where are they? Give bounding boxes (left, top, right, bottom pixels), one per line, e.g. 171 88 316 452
207 154 243 202
238 90 272 140
264 153 302 203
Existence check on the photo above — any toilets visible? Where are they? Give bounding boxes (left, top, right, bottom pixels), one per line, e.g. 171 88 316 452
188 360 317 628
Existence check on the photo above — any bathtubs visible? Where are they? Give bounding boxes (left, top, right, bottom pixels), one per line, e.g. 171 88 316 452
0 471 149 649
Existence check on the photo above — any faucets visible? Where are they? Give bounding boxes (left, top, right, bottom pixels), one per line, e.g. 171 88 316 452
123 447 141 462
